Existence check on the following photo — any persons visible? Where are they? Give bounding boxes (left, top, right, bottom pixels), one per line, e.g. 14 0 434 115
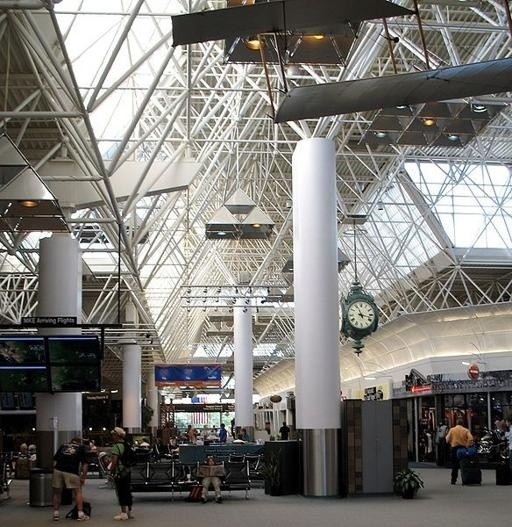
18 443 28 460
27 444 37 461
53 427 151 521
424 431 433 457
494 416 512 451
434 422 449 467
264 422 270 435
185 418 249 443
445 418 473 486
279 422 290 440
201 458 223 504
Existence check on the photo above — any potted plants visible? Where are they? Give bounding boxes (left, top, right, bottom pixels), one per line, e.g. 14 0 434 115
395 466 425 499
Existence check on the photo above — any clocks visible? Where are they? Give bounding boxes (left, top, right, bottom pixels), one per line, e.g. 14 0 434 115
341 217 380 358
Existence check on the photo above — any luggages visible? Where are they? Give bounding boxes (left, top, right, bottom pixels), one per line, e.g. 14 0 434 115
460 457 481 486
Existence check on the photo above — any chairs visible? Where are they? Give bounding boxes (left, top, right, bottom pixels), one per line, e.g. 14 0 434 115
2 426 269 504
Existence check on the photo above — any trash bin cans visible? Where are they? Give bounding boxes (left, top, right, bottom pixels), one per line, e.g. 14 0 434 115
29 467 53 507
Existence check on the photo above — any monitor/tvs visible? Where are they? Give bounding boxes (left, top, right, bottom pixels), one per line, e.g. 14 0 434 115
47 365 101 392
46 335 100 365
0 366 47 392
0 336 46 366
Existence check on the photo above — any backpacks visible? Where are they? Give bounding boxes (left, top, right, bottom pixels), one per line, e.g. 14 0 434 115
115 442 138 468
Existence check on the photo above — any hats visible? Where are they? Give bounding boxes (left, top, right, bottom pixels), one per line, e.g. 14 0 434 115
114 427 125 437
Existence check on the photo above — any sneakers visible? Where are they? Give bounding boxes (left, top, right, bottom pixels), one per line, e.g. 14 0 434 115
113 513 128 520
53 513 59 520
77 514 90 521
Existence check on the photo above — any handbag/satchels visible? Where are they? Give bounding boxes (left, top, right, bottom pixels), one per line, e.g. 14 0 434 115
66 502 91 519
190 486 201 502
457 448 475 459
